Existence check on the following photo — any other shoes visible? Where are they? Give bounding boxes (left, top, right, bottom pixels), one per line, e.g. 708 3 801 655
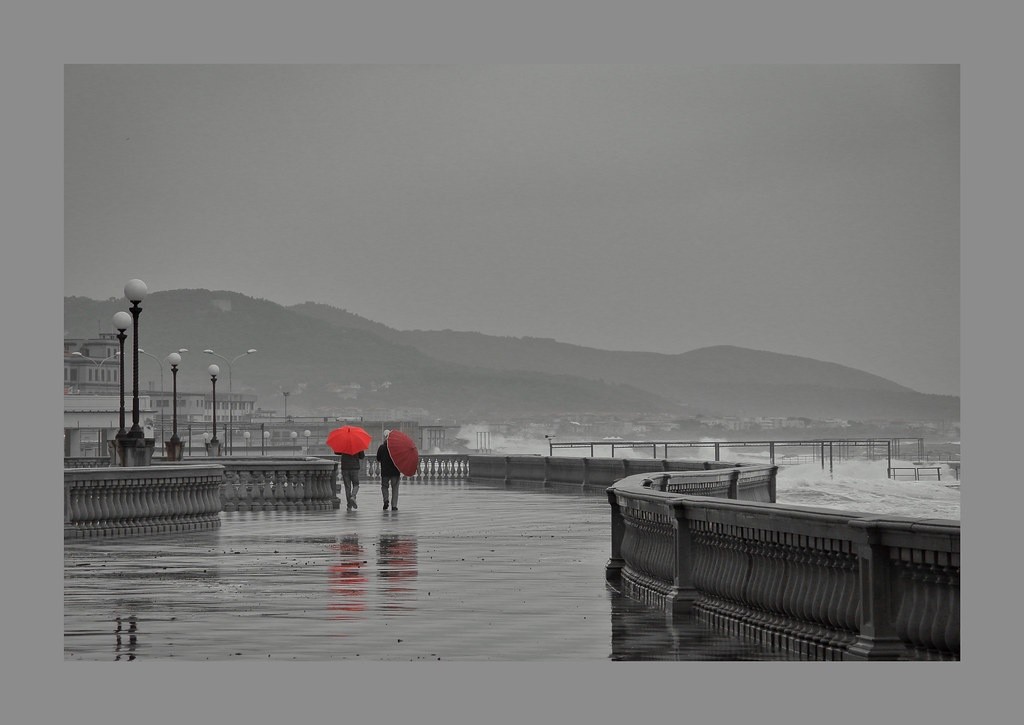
347 500 353 509
350 497 357 509
392 507 398 510
383 503 389 510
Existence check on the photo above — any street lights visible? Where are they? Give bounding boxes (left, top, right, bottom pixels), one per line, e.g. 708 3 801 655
290 431 298 455
244 432 250 455
166 351 183 443
303 430 311 455
203 349 256 455
124 279 147 439
264 431 270 455
139 347 189 456
209 364 221 442
111 310 131 437
72 351 127 455
202 433 209 455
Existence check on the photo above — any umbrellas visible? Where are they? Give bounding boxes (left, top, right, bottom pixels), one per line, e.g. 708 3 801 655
387 428 419 476
327 425 372 455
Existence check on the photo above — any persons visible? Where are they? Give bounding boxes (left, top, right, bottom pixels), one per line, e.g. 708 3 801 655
334 450 366 511
376 430 401 511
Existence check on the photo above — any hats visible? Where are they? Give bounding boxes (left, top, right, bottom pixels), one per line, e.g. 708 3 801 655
383 430 390 439
